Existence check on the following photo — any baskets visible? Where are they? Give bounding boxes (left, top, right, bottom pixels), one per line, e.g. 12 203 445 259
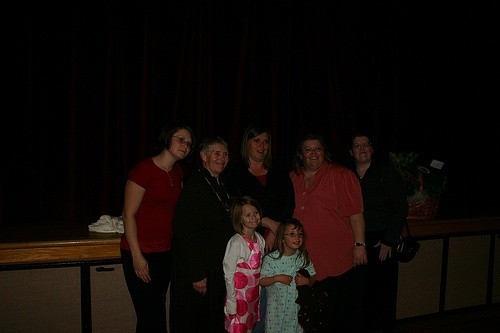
404 170 439 220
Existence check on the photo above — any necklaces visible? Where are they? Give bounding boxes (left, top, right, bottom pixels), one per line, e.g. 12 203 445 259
159 164 173 182
356 166 369 180
198 168 231 212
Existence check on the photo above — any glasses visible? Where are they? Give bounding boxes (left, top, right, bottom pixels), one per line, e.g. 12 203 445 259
285 233 304 238
352 142 371 148
301 146 322 152
172 136 193 148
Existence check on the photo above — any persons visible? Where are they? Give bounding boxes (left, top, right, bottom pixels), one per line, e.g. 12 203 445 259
259 218 317 333
229 123 408 333
223 195 266 333
170 134 237 333
119 124 197 333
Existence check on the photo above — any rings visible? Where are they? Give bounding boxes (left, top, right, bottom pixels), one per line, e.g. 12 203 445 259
381 255 384 258
138 272 141 275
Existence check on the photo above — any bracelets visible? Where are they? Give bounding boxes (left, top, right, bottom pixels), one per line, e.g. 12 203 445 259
352 241 366 248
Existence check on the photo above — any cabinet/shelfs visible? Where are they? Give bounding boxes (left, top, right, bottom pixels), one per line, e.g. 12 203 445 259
0 223 500 333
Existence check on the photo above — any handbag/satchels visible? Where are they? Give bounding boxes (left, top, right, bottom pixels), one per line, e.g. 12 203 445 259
397 235 420 263
